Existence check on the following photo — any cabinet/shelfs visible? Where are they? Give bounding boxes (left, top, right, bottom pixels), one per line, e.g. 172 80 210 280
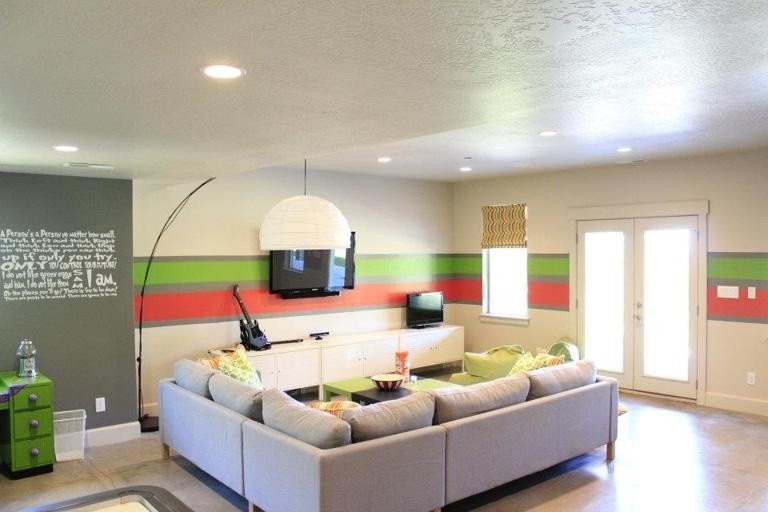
0 369 54 478
220 323 466 401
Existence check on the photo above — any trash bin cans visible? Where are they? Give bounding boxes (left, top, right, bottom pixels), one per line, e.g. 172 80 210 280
52 409 87 463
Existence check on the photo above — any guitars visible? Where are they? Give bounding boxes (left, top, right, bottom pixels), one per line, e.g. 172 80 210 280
233 283 269 350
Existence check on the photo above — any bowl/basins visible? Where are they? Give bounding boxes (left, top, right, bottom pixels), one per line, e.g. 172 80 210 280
370 374 404 393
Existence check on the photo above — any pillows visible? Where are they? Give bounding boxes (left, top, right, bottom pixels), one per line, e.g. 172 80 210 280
448 337 579 386
198 344 264 389
307 401 360 419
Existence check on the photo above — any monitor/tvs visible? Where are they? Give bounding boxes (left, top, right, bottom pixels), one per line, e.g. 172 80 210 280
270 231 356 299
407 292 444 329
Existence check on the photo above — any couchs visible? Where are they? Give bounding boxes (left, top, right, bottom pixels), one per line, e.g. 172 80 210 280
156 375 619 512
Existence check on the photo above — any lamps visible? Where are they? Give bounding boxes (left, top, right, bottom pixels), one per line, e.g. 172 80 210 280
258 159 353 251
